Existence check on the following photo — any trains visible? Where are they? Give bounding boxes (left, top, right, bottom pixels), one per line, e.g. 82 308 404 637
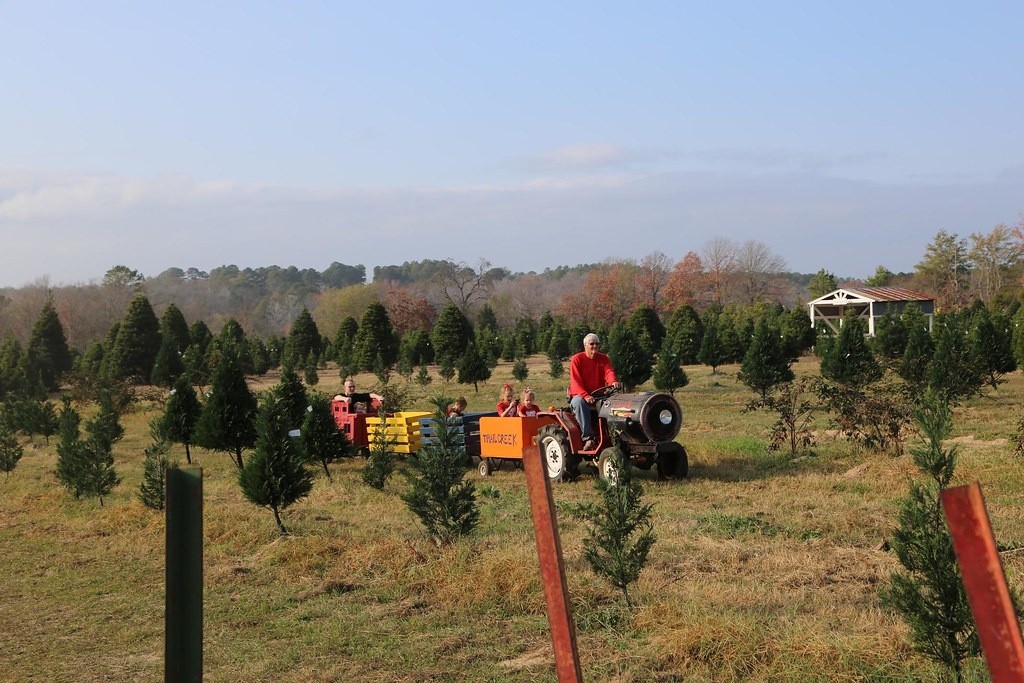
324 384 690 492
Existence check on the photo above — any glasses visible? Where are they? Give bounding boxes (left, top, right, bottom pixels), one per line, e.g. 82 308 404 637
587 342 600 346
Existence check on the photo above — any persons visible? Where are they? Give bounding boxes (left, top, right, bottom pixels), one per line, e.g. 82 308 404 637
515 387 542 417
444 397 467 426
496 384 521 417
333 379 385 414
353 402 364 414
570 333 621 451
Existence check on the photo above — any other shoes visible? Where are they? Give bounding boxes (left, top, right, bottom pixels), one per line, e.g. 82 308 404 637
583 439 598 451
